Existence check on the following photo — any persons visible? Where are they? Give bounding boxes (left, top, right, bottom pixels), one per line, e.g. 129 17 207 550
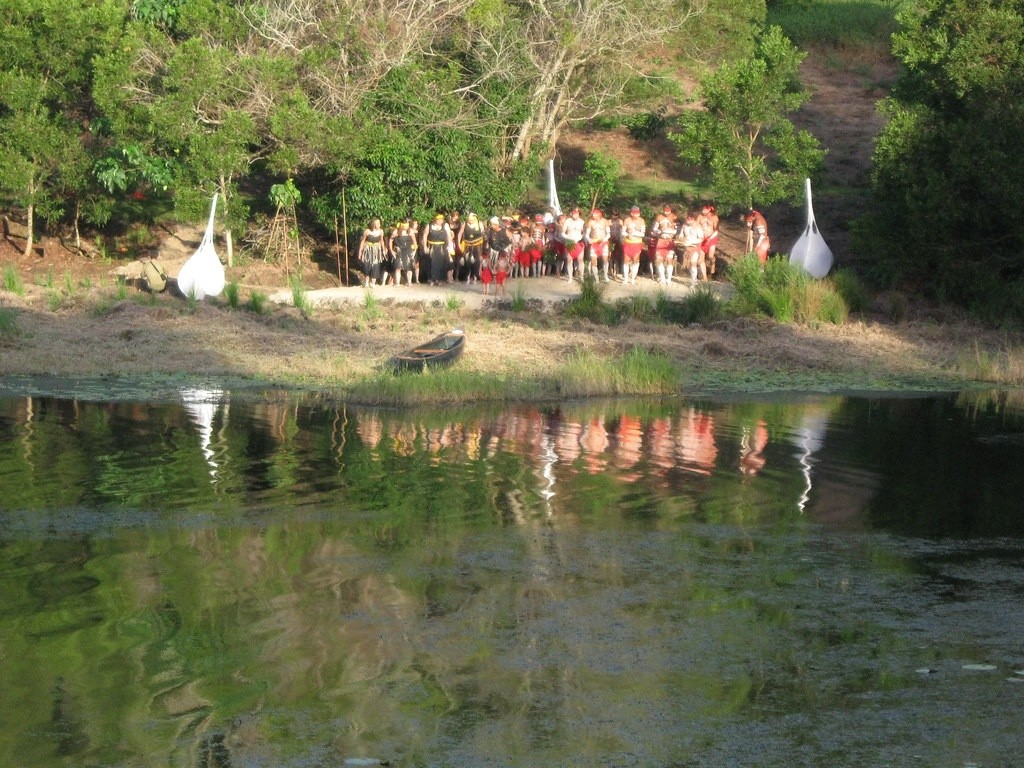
745 208 771 262
356 203 719 300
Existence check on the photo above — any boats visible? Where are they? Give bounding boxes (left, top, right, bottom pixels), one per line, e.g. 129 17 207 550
175 194 227 304
391 324 466 374
543 157 564 225
785 177 834 281
